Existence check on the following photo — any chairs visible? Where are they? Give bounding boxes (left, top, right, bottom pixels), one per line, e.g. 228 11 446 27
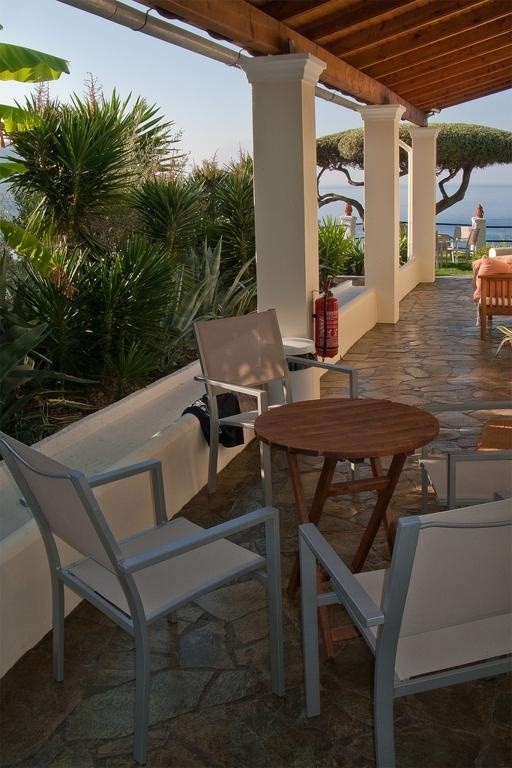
2 430 283 762
297 497 511 767
478 265 512 342
448 226 470 266
193 309 356 507
419 448 511 516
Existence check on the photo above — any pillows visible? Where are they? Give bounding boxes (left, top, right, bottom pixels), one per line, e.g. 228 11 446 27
474 255 510 302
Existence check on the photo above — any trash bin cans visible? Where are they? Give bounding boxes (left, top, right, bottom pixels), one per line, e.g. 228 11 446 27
262 336 320 403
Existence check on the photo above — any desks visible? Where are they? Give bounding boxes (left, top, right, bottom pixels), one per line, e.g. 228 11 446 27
255 396 440 649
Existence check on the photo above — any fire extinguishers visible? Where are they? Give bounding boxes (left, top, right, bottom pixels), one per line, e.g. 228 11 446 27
314 275 339 357
